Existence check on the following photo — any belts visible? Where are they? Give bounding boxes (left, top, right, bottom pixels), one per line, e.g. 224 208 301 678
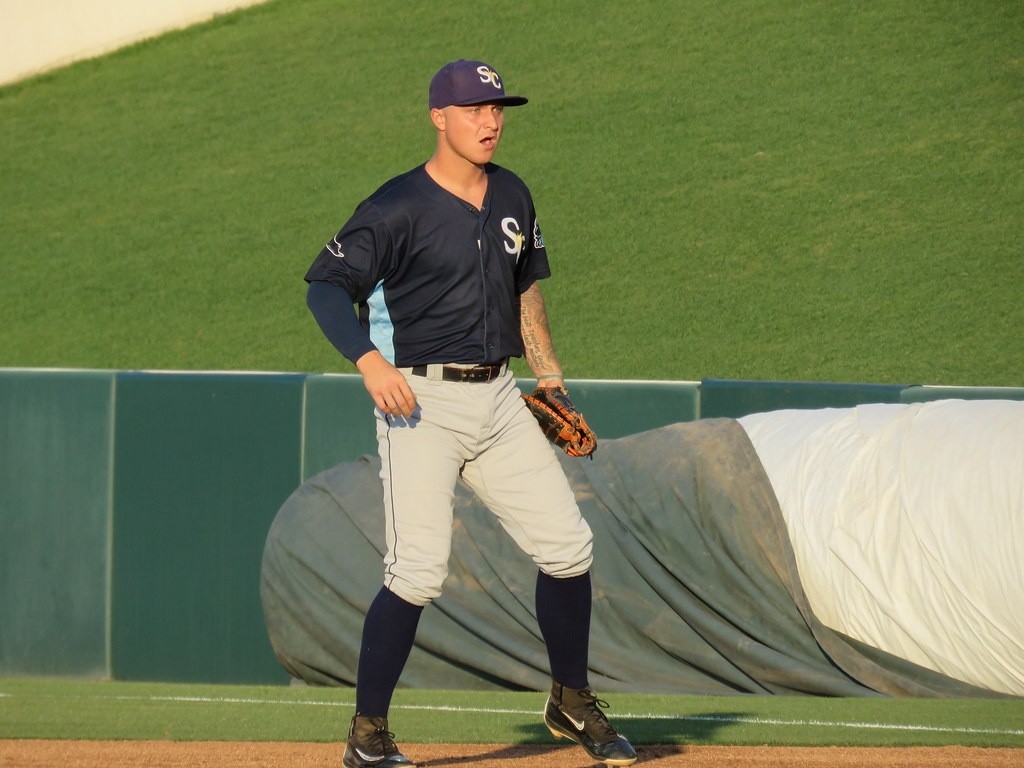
412 363 501 383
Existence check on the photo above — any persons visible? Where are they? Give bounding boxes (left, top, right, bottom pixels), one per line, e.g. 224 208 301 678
304 59 638 767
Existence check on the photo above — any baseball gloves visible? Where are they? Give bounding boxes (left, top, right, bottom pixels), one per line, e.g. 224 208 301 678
520 385 600 461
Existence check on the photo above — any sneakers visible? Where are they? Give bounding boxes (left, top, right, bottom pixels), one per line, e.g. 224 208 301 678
343 713 417 768
543 678 638 767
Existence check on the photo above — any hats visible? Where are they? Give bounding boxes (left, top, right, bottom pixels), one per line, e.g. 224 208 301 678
428 59 528 111
